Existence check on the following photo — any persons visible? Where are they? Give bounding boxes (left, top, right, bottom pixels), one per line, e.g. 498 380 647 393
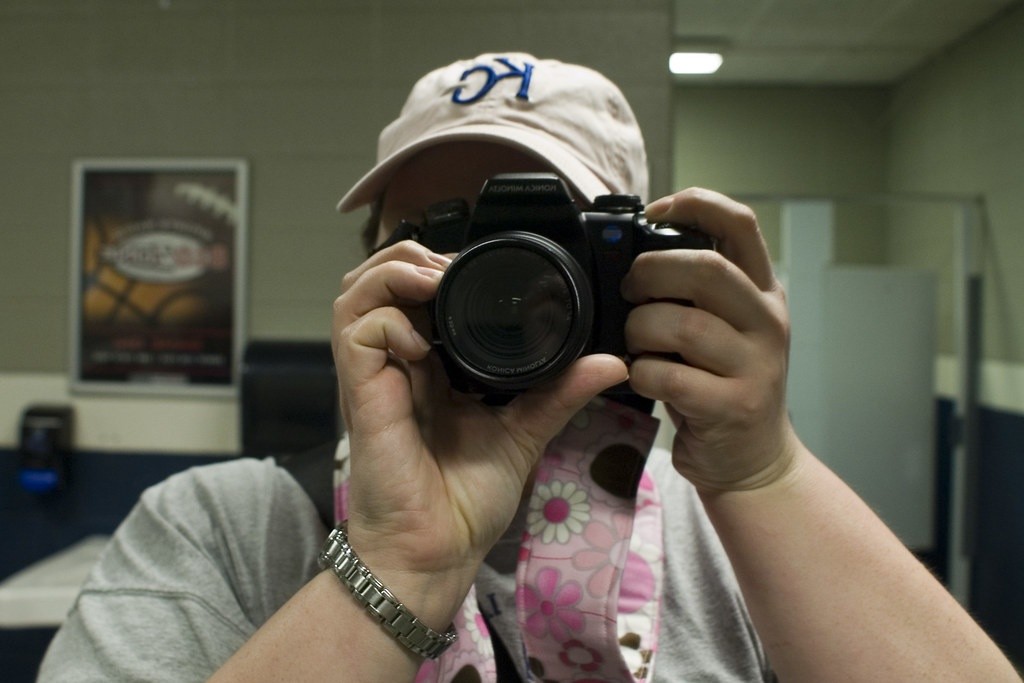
37 52 1024 683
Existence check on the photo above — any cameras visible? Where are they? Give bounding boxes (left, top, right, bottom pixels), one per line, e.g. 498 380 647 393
413 172 716 399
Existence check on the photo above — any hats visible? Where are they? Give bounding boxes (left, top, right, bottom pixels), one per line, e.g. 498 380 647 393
337 53 651 219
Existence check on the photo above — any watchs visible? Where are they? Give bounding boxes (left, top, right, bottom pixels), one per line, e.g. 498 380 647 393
316 517 458 661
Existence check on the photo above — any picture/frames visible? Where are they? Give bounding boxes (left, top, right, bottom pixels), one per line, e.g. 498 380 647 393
69 155 248 399
